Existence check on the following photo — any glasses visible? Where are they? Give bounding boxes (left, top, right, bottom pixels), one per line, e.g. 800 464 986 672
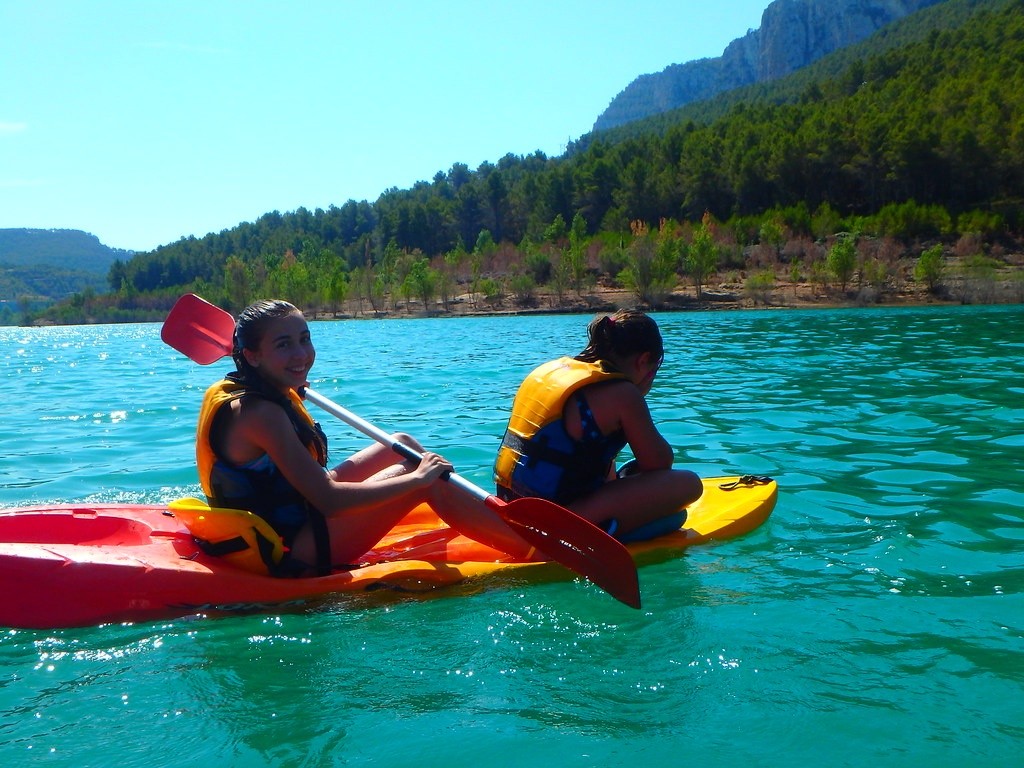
720 475 773 490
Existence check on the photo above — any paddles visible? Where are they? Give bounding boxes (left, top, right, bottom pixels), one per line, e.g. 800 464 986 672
159 291 646 608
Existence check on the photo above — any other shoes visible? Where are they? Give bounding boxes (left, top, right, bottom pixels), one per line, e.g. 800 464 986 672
608 509 687 545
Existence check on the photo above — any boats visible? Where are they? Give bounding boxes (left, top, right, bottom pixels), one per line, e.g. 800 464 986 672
1 471 784 626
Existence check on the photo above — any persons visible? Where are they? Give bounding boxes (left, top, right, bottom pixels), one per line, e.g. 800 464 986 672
196 299 555 579
493 308 703 535
